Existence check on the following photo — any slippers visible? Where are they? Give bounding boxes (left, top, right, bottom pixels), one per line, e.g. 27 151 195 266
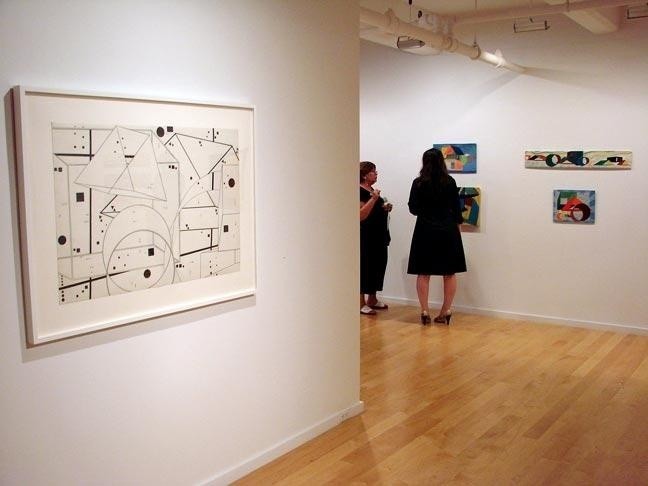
360 304 377 315
368 300 388 309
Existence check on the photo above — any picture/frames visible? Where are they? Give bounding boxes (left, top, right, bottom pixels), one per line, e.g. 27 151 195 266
12 85 257 350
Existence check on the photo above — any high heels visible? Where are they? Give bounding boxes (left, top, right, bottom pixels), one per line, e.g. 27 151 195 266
421 310 431 325
434 309 452 325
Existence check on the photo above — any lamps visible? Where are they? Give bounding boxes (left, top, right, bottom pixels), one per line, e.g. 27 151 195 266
626 4 648 21
513 20 550 34
393 36 426 50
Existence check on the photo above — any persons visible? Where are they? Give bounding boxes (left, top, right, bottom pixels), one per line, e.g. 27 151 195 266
360 162 392 315
407 148 467 325
